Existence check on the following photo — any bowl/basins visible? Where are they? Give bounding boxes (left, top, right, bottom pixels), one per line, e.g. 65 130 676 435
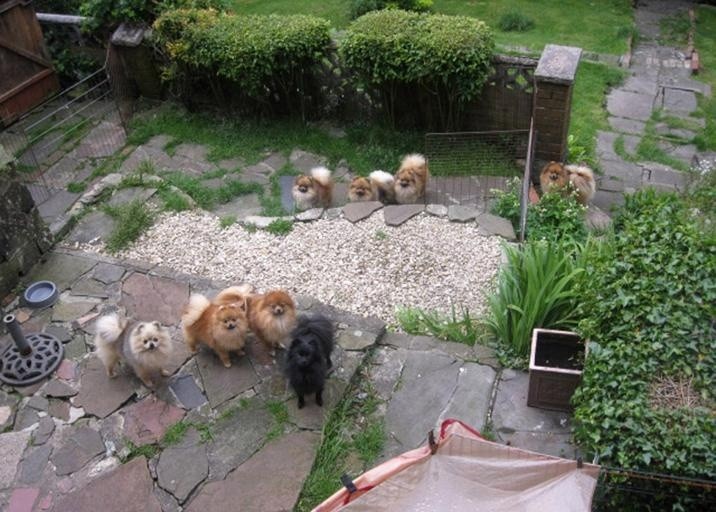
24 280 59 309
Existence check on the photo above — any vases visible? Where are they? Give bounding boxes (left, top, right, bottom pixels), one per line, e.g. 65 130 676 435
525 327 589 412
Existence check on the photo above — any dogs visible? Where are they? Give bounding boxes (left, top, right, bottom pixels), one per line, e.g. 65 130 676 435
180 283 250 367
93 310 173 389
536 160 597 207
281 312 339 410
346 152 430 204
220 285 297 358
289 166 334 210
